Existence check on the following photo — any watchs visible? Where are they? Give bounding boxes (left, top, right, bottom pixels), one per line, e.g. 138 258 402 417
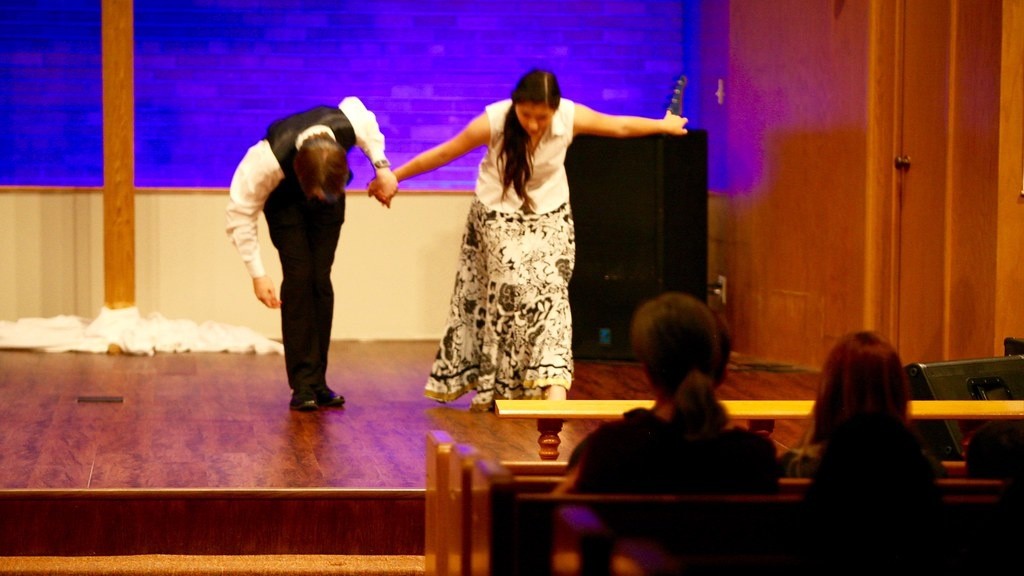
372 158 392 170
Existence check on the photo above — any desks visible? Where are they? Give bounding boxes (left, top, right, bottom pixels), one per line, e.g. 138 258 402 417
497 400 1024 461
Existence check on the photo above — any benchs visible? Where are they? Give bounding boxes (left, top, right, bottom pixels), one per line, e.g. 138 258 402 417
427 430 1016 576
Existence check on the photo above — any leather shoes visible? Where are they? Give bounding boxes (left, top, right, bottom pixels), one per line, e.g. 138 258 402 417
289 387 346 413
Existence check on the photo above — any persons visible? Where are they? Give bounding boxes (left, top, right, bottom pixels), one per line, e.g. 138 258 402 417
224 95 398 411
778 330 951 500
367 70 688 418
548 292 777 497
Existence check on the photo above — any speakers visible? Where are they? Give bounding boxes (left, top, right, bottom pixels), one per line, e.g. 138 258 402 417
905 355 1021 461
566 128 708 359
